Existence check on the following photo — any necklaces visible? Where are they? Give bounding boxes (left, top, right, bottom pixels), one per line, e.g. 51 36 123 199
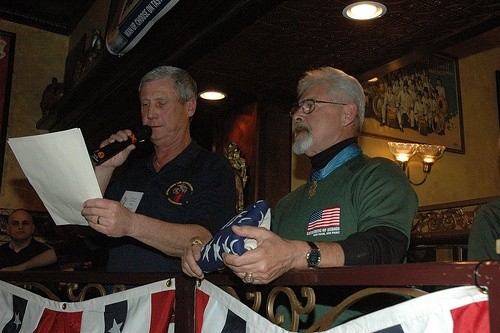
305 180 318 200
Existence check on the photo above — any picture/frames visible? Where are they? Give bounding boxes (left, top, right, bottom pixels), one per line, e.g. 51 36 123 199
0 29 16 188
362 53 465 156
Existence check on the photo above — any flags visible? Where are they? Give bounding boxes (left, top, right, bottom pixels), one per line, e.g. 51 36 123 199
0 277 176 333
193 277 490 333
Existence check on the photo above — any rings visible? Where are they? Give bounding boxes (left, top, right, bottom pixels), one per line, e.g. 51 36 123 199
191 240 203 246
97 216 101 224
244 272 255 284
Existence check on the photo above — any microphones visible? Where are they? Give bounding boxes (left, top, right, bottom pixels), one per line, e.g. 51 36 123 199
89 123 152 165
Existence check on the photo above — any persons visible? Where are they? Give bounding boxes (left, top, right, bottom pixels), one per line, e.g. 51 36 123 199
182 66 419 333
369 67 447 136
81 65 237 296
0 208 63 297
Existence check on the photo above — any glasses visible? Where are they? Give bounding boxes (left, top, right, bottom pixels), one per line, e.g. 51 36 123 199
289 99 359 117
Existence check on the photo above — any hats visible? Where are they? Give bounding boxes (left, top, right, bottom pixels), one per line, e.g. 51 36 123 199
432 89 439 95
387 81 393 87
423 87 428 93
416 90 422 96
394 76 400 82
403 80 409 87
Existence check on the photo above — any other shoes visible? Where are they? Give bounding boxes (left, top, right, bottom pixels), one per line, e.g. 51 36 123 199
400 128 403 132
380 122 384 126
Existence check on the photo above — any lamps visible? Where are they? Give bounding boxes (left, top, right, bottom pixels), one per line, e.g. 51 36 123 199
386 141 447 185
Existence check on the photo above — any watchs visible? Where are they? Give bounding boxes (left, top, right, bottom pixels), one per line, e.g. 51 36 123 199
305 240 321 270
